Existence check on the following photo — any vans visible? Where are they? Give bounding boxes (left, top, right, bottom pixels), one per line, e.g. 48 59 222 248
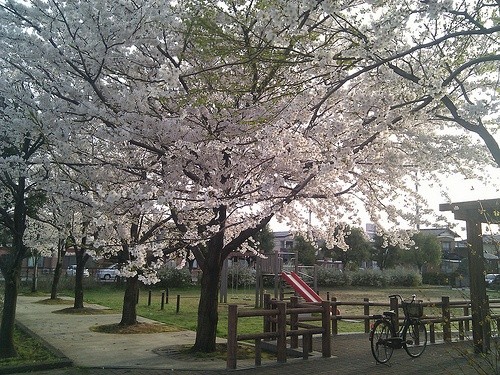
485 273 500 290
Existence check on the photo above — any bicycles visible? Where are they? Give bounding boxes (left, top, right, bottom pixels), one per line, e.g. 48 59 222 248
370 292 428 367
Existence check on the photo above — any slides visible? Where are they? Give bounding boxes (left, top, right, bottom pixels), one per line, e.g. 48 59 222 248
282 269 340 319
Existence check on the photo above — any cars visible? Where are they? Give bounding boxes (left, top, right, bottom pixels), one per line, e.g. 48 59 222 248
66 264 90 278
98 262 126 280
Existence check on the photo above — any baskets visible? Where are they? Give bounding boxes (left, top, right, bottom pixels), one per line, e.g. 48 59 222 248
403 300 424 318
